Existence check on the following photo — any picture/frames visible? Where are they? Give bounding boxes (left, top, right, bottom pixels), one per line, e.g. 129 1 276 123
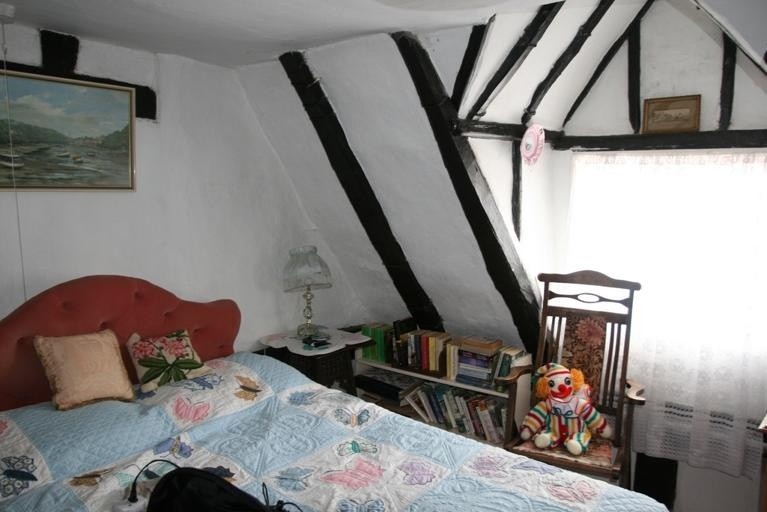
642 94 702 136
0 68 138 193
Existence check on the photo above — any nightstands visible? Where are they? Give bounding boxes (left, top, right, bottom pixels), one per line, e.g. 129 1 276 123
272 324 376 397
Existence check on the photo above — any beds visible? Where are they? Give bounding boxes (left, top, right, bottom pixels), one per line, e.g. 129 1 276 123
1 276 670 512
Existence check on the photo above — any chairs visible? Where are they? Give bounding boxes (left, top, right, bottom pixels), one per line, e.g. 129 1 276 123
503 271 646 491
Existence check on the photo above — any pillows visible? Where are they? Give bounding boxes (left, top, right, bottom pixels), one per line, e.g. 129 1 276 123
126 329 216 393
32 329 135 411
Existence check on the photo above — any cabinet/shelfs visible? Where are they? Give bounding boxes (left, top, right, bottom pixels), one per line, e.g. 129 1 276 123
357 349 533 449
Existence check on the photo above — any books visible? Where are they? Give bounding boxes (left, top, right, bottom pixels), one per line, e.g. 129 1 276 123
351 365 509 445
361 315 525 394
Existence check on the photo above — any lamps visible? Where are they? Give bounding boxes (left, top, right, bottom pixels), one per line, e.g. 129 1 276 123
283 245 332 336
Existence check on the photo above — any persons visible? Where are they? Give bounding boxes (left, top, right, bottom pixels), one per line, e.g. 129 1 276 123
518 361 613 457
